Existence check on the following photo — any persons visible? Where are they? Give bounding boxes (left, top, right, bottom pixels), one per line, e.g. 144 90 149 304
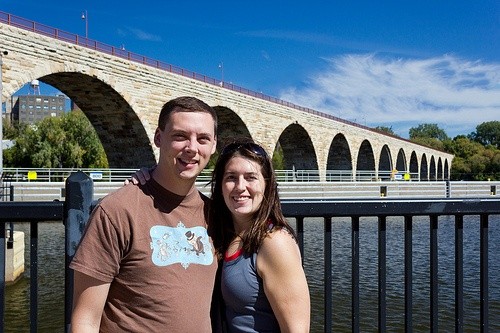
124 142 310 333
70 96 224 333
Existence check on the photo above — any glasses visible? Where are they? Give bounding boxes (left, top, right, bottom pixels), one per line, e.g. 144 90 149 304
220 141 267 159
